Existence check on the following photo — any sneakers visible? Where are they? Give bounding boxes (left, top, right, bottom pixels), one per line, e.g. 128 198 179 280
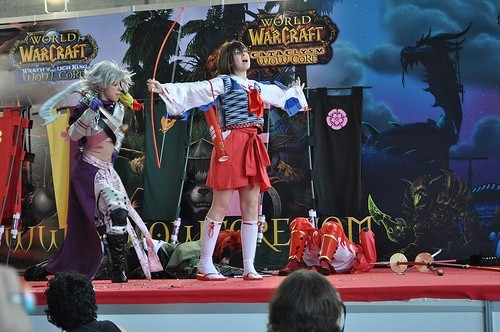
320 260 330 274
278 260 300 276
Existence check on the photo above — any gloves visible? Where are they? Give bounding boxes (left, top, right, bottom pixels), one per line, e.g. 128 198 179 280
112 150 118 160
89 98 104 112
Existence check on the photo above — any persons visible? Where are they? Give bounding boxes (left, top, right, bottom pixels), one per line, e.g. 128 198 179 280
45 273 122 332
148 41 306 280
279 217 376 275
25 230 263 281
44 61 164 283
269 269 346 332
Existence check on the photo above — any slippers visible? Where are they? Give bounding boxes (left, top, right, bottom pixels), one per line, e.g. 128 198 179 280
197 273 227 281
243 272 263 280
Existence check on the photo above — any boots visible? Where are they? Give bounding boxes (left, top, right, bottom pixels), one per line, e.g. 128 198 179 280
107 232 128 282
24 261 54 281
